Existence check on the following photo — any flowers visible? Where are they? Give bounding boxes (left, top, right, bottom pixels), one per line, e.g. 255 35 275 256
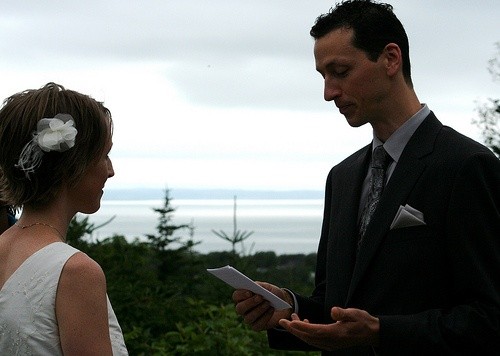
14 114 78 181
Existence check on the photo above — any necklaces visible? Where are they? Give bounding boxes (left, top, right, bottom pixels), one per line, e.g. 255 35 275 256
14 222 67 243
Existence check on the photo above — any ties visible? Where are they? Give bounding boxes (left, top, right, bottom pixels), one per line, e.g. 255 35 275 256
357 145 391 263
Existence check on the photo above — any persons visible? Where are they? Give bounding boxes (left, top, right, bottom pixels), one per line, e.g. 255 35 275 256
0 83 130 356
232 0 500 356
0 201 19 235
